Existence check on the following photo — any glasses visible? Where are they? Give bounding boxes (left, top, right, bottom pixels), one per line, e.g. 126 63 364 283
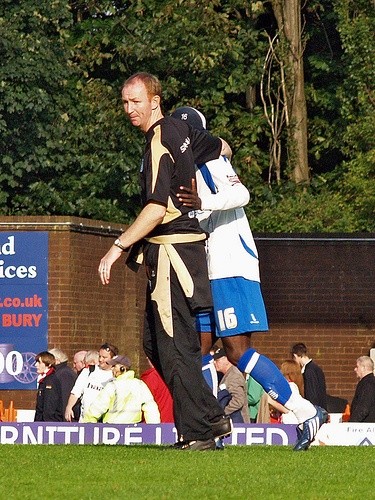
102 344 116 355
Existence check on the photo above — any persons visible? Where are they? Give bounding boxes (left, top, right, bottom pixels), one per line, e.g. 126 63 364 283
349 355 375 422
97 72 232 451
34 343 329 424
170 106 328 451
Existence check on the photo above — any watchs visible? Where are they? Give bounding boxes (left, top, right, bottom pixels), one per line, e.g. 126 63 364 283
114 238 125 251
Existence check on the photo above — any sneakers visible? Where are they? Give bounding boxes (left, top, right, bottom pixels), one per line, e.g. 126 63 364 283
172 437 216 451
293 405 328 450
209 415 232 440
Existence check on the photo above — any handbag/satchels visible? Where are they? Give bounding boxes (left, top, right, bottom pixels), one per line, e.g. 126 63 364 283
270 411 283 424
71 398 82 422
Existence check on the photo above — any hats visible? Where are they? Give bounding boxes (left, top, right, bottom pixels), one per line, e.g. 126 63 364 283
212 348 226 358
169 106 207 132
106 354 132 368
